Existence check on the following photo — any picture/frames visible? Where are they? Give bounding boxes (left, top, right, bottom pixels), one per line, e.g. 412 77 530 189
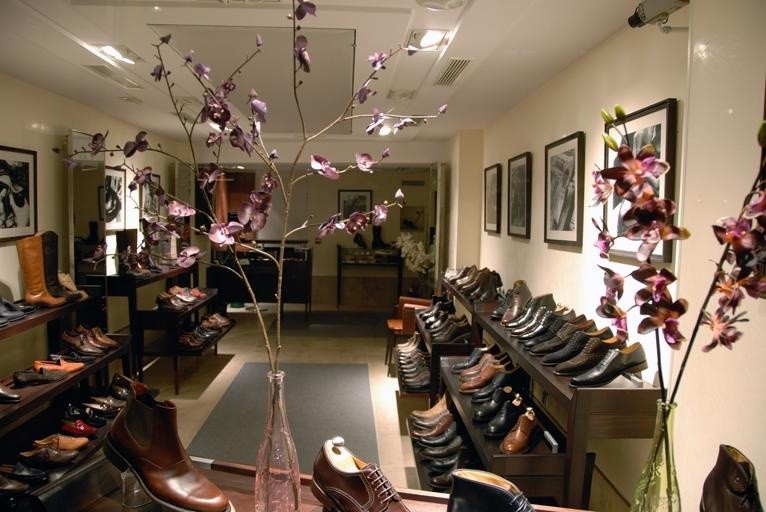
600 97 680 265
336 189 373 225
0 146 41 243
484 162 503 234
138 172 162 230
103 166 127 234
506 151 532 240
543 128 586 247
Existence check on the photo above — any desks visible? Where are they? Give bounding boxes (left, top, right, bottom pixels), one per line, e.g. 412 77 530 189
205 240 314 320
334 244 404 310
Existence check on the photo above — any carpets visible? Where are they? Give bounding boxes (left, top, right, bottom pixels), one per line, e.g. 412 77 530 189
185 359 382 477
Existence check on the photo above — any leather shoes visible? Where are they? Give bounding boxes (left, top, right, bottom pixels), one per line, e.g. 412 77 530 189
172 310 233 353
308 435 414 511
153 279 211 311
1 371 150 501
450 343 542 454
418 289 471 346
448 264 503 303
0 321 121 403
410 392 480 490
394 330 431 393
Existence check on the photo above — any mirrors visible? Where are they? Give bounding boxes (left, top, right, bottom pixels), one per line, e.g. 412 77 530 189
63 127 113 401
426 159 446 297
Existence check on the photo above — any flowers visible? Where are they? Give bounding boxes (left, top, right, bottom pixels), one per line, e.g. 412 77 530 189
48 0 452 506
584 99 766 511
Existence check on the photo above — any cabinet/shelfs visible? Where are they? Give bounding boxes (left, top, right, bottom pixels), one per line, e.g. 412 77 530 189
405 302 668 510
18 440 586 511
391 274 515 399
80 256 237 399
0 292 160 503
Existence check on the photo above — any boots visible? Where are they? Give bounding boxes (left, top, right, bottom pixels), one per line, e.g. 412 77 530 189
0 223 86 327
100 377 240 511
698 444 761 512
490 281 648 386
446 462 541 512
115 228 153 280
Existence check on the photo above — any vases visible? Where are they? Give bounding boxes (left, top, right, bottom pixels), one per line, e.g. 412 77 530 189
254 369 299 511
630 398 683 510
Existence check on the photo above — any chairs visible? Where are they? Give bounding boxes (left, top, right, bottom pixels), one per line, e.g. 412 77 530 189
385 286 442 375
